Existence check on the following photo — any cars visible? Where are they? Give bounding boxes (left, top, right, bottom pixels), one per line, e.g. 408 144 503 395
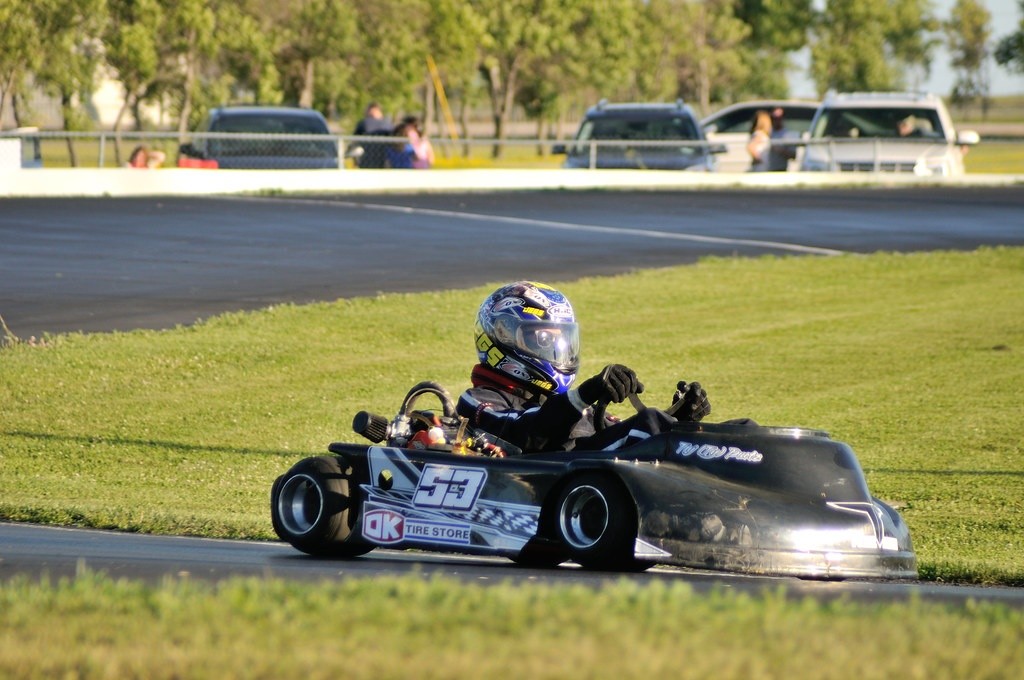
698 97 823 172
798 84 981 177
550 99 727 170
180 107 367 169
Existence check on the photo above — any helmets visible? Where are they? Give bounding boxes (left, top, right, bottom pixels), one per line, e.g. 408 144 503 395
474 281 581 395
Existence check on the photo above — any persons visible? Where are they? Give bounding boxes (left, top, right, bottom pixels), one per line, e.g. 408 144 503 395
384 124 415 168
126 146 166 168
897 114 925 138
747 109 784 173
349 103 393 168
455 281 711 452
401 115 434 169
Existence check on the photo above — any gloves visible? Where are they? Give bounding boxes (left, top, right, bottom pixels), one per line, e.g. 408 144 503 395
672 381 711 422
578 363 644 407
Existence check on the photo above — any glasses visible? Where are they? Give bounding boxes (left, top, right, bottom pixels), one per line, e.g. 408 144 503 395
522 329 557 349
